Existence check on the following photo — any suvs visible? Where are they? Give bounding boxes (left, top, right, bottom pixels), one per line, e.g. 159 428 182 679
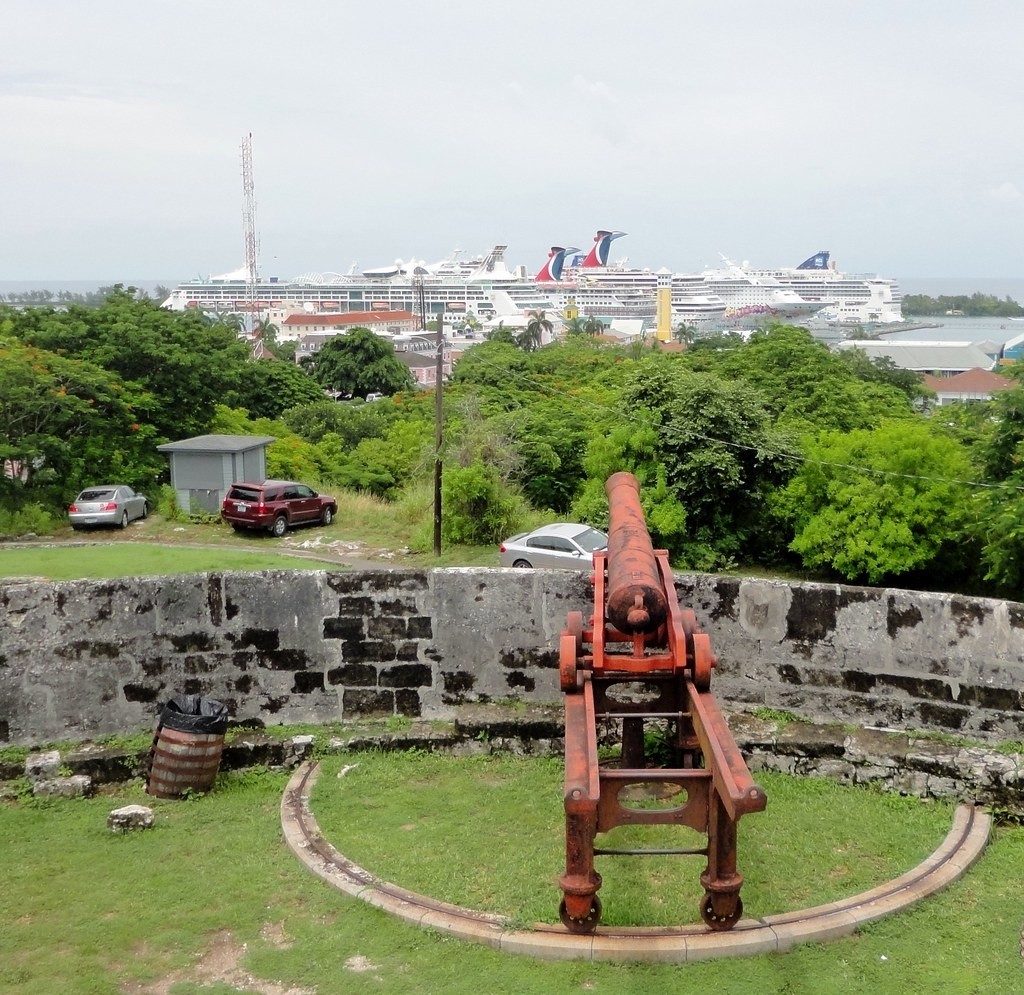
221 480 338 538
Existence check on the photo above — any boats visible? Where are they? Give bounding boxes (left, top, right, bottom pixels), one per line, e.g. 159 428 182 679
158 239 904 328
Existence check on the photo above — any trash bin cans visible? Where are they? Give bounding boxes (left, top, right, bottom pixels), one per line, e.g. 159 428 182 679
146 697 227 800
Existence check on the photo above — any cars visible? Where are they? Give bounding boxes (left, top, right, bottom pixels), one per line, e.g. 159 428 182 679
67 484 150 530
499 523 609 568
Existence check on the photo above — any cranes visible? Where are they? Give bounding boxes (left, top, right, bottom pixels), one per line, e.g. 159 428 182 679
240 133 265 360
